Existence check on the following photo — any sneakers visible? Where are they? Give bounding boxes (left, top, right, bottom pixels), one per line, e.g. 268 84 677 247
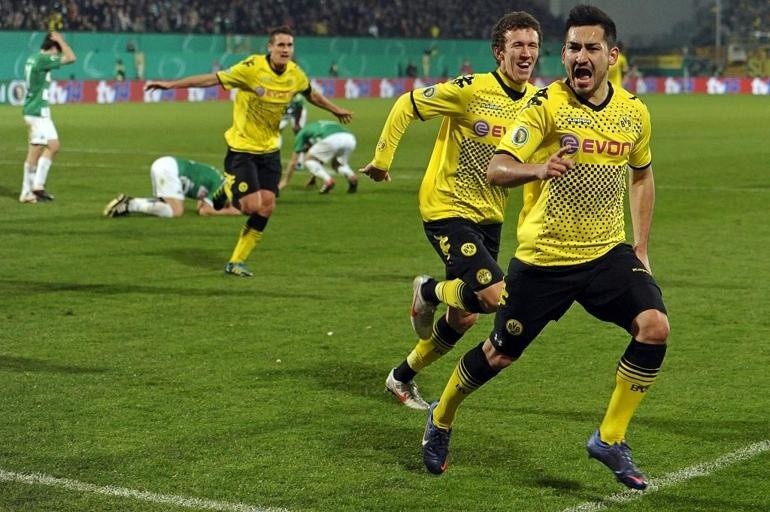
320 179 335 193
347 177 358 193
410 274 432 341
385 367 430 411
226 262 252 276
586 431 647 490
103 193 133 218
422 402 452 474
212 182 229 210
19 188 53 203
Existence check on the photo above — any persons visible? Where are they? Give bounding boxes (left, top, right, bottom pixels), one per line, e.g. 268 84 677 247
330 58 338 77
277 119 360 194
103 157 239 219
144 28 353 277
19 31 77 204
217 35 250 103
398 53 474 79
287 95 301 130
359 12 544 411
422 1 669 489
1 0 565 39
609 43 627 87
113 61 125 78
616 0 770 53
